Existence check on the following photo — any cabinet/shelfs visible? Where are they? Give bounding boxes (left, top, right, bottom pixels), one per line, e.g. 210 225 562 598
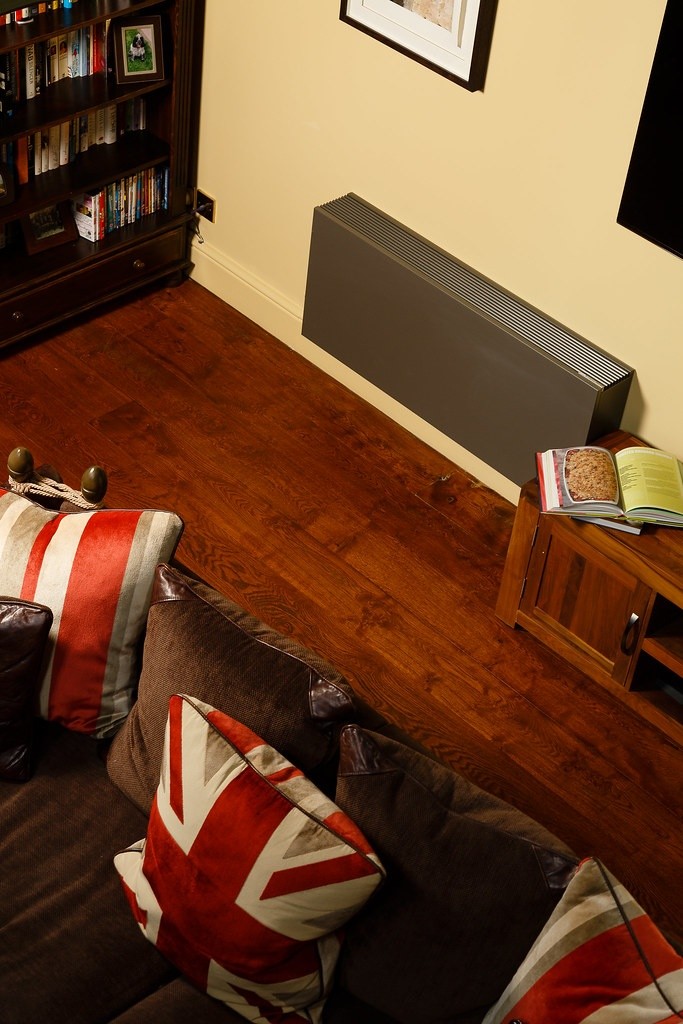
0 0 206 350
494 429 683 749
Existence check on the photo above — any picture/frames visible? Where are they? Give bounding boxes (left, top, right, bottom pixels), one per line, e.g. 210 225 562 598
113 14 165 84
339 0 498 93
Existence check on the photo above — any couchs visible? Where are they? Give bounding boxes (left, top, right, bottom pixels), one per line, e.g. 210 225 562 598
0 447 683 1024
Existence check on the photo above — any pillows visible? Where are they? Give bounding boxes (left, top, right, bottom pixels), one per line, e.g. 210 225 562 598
112 694 387 1023
0 594 54 785
0 486 184 739
480 855 683 1024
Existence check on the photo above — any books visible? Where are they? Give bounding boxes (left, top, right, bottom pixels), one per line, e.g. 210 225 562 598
71 166 168 243
535 447 683 535
0 0 78 25
0 19 116 123
0 95 147 186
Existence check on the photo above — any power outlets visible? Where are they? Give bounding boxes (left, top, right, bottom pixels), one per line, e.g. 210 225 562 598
196 189 215 222
